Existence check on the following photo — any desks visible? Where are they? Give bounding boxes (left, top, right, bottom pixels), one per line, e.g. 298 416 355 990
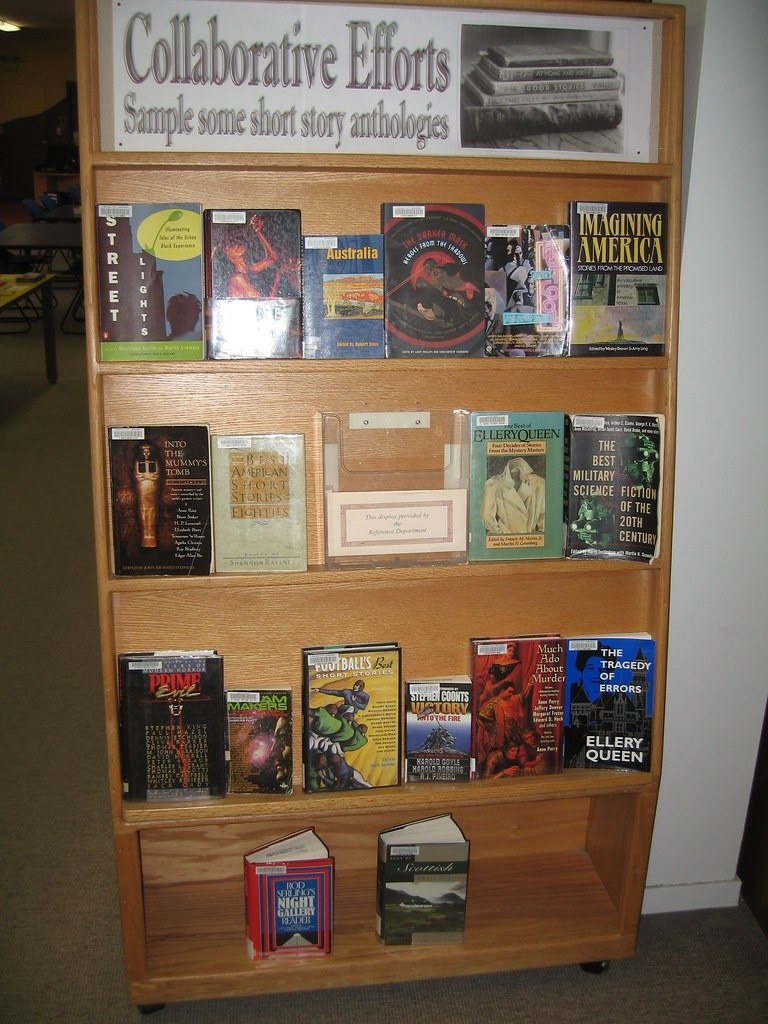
32 170 79 204
0 222 82 383
31 205 81 220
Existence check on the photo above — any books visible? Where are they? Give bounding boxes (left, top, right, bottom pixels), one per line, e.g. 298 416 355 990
111 630 655 803
108 409 666 576
243 826 335 959
460 43 622 138
374 813 471 947
94 198 667 361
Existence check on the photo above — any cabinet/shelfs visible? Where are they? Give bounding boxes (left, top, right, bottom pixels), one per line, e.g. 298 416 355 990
75 0 688 1017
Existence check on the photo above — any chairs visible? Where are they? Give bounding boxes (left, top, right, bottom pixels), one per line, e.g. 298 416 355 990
21 192 72 224
